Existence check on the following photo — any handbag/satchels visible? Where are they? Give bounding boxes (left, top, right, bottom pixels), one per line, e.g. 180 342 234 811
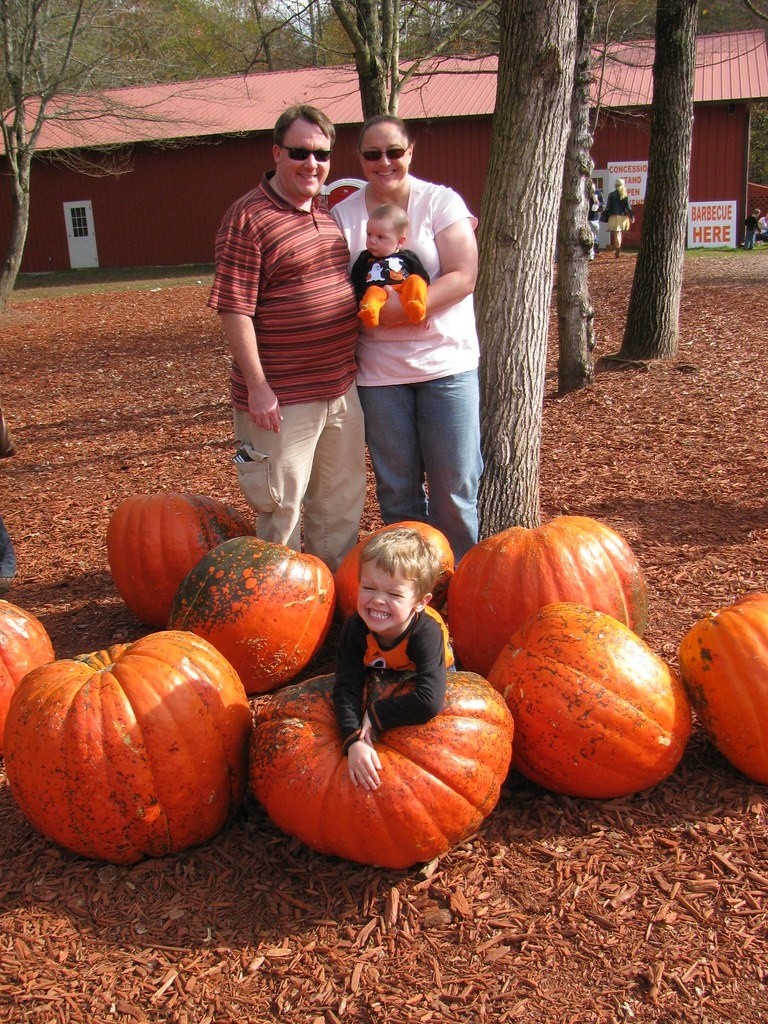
600 209 609 223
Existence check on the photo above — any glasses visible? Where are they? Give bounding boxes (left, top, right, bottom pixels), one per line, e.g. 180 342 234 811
282 144 333 162
360 145 409 162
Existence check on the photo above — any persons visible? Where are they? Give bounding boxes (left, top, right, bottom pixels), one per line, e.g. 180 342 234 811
329 115 484 565
332 526 456 791
743 209 768 250
606 179 635 259
207 106 367 574
587 183 606 261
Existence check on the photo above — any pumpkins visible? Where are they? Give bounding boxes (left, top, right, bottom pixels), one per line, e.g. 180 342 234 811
446 516 648 678
0 597 56 755
168 535 335 697
247 666 515 868
3 629 256 863
105 494 260 629
485 602 692 801
327 521 454 646
680 592 768 789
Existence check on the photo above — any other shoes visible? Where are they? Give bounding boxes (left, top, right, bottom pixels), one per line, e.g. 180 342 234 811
587 250 594 261
748 247 753 250
615 248 620 258
